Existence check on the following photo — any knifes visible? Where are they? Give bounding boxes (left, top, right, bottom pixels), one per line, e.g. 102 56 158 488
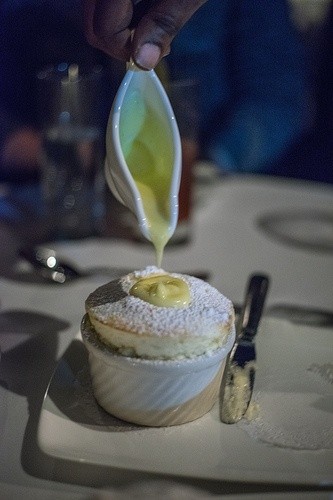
221 272 271 423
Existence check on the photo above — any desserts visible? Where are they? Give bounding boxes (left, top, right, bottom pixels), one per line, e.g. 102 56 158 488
80 265 237 425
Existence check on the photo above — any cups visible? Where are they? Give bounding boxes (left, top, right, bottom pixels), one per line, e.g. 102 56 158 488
81 314 236 427
103 28 182 244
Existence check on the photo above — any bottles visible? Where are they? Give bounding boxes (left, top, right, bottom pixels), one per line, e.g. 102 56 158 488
133 55 194 246
36 61 100 241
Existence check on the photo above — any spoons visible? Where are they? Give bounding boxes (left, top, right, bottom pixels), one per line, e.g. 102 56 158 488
16 246 208 286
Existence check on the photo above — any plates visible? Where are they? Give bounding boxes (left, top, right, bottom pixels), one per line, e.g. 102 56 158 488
36 315 333 488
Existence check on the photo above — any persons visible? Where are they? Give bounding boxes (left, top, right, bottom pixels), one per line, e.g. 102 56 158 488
0 0 318 178
76 0 212 71
